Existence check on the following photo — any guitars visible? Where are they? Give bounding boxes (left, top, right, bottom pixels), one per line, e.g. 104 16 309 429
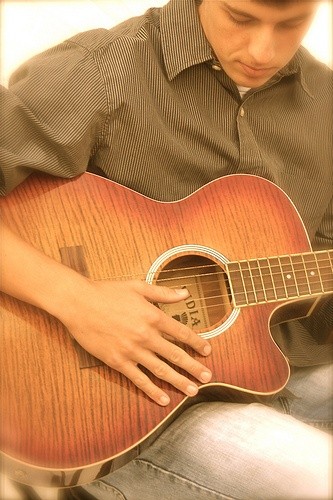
0 165 333 490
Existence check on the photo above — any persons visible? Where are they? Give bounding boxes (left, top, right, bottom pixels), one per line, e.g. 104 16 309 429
0 1 333 499
